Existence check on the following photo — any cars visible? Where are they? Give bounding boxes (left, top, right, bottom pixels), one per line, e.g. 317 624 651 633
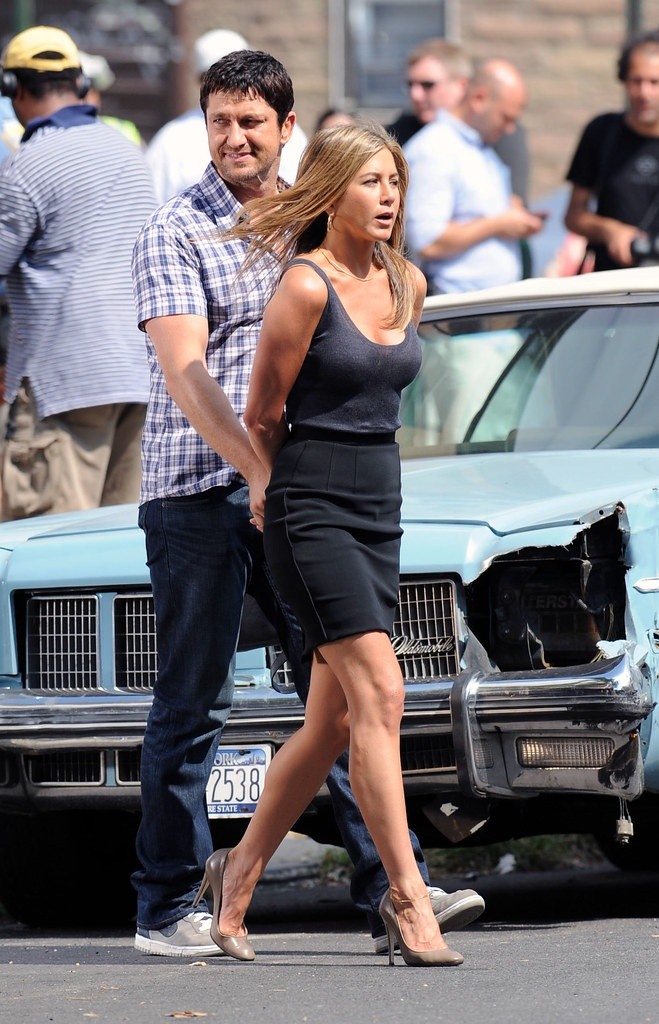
0 266 659 919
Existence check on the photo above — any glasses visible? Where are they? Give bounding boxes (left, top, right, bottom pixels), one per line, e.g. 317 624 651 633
404 80 435 91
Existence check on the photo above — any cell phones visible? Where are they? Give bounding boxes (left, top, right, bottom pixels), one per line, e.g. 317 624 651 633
528 210 551 218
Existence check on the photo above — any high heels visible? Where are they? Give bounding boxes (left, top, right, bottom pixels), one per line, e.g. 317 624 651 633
192 848 256 960
378 889 463 965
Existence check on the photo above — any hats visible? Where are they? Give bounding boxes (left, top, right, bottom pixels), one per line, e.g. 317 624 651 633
191 28 250 76
4 25 81 72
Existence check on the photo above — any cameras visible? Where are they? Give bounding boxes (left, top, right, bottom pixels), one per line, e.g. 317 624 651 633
630 236 659 268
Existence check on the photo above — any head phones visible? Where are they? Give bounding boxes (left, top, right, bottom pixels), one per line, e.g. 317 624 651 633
0 74 91 99
617 32 659 81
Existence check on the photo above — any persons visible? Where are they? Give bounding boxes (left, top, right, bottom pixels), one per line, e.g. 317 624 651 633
132 51 486 967
0 28 308 523
381 39 546 447
564 31 659 277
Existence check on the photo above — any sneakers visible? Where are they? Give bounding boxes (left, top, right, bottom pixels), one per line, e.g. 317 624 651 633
134 912 225 956
371 887 486 952
